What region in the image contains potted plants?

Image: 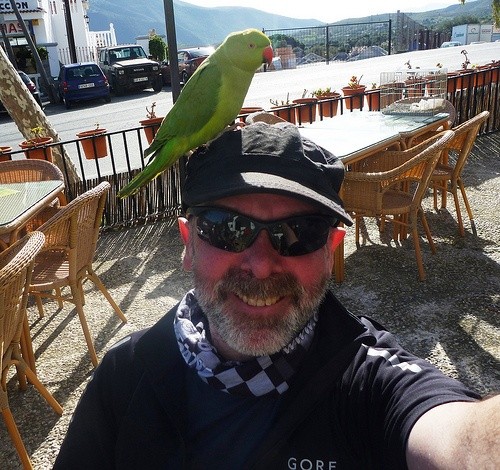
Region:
[269,93,297,125]
[139,101,165,146]
[294,89,317,123]
[311,87,340,117]
[76,123,109,160]
[20,125,54,164]
[366,83,382,111]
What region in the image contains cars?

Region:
[160,48,218,85]
[0,70,44,118]
[439,41,461,48]
[58,63,111,110]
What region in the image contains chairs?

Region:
[0,231,63,470]
[335,130,455,283]
[0,180,65,234]
[0,160,66,244]
[380,96,456,132]
[406,110,490,237]
[15,181,128,384]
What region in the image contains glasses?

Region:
[186,204,339,256]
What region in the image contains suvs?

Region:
[99,45,163,96]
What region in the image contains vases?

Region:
[342,86,366,109]
[456,70,475,89]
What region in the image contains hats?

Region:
[179,120,354,227]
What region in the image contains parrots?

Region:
[114,28,273,201]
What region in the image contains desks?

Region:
[271,111,449,210]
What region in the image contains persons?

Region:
[53,121,500,470]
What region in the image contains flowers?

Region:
[459,50,472,68]
[347,76,358,83]
